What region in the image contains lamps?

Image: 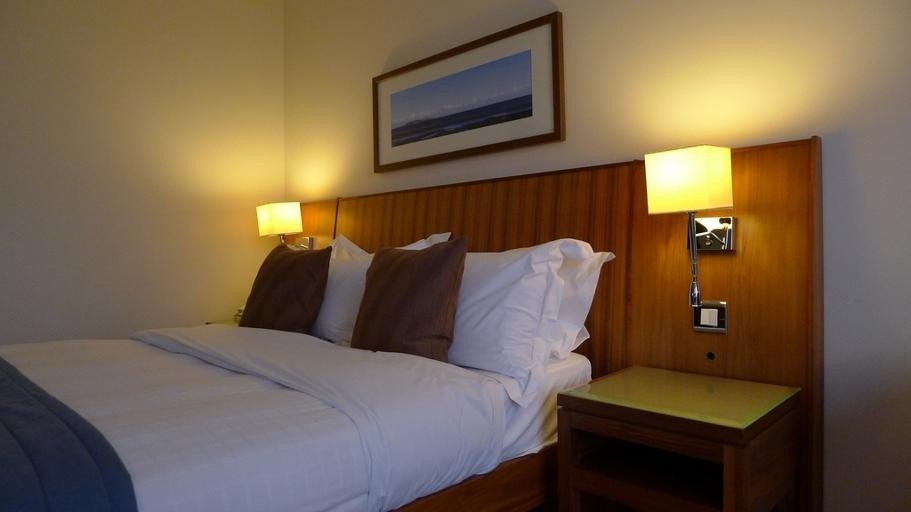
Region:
[642,143,735,312]
[255,201,312,253]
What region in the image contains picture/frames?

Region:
[371,10,566,173]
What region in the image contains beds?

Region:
[0,323,593,512]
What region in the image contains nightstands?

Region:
[551,354,801,512]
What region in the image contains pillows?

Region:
[310,236,450,341]
[236,243,333,334]
[449,239,618,408]
[350,236,466,363]
